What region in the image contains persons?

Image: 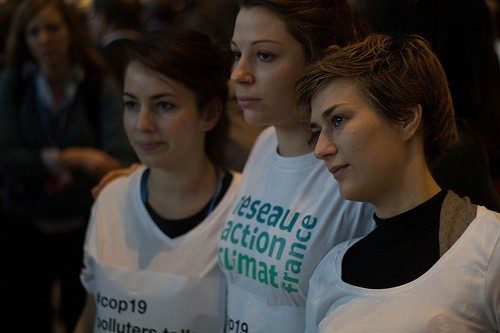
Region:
[0,1,242,333]
[215,0,376,333]
[297,28,500,333]
